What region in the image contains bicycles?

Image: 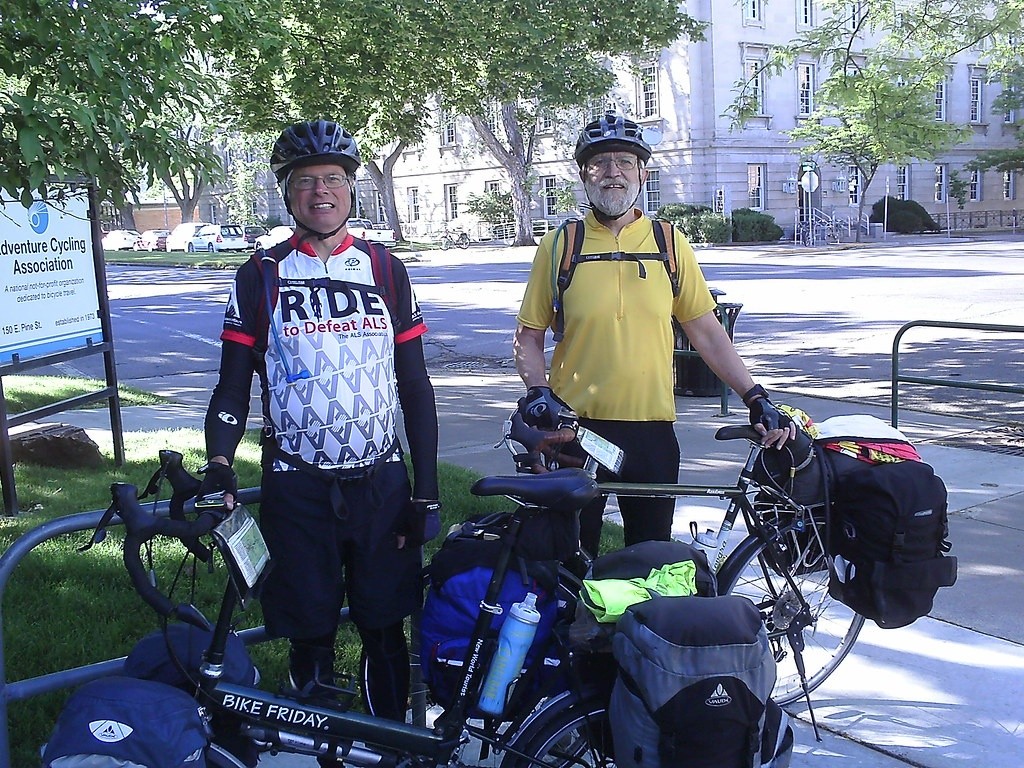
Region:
[77,447,618,768]
[359,424,867,746]
[438,229,470,250]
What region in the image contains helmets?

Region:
[575,116,652,170]
[270,120,362,183]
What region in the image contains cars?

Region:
[99,229,142,249]
[184,224,249,253]
[165,223,212,253]
[254,226,296,251]
[133,230,171,253]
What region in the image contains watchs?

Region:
[742,384,770,405]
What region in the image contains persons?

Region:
[197,118,440,768]
[514,114,796,559]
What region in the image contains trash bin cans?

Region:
[670,302,743,397]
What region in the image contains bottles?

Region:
[475,593,540,714]
[688,521,720,571]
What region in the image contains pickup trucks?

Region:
[345,218,396,249]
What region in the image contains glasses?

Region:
[290,174,350,190]
[585,156,638,170]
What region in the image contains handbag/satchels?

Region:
[41,675,212,768]
[608,595,793,768]
[753,447,869,577]
[128,623,261,768]
[828,460,957,629]
[570,540,718,757]
[420,511,592,729]
[775,403,922,464]
